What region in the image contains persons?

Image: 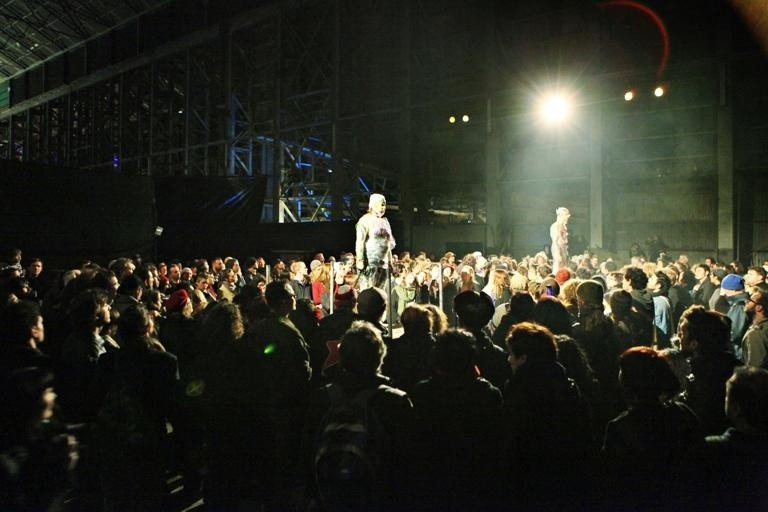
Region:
[355,192,397,289]
[1,234,768,511]
[549,206,571,274]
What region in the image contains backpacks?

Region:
[313,383,380,510]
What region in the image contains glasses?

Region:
[746,297,757,304]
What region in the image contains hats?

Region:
[576,280,603,304]
[165,289,187,311]
[359,287,385,314]
[452,291,494,326]
[720,275,746,290]
[611,290,633,313]
[332,284,357,307]
